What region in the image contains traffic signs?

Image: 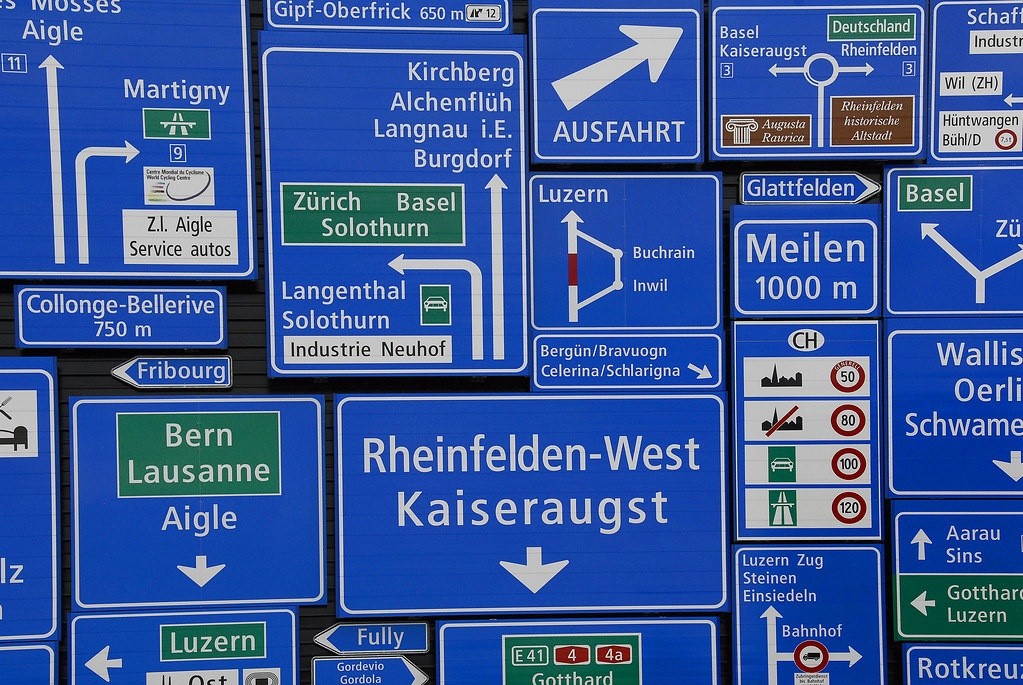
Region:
[67,393,328,613]
[528,171,727,393]
[261,0,513,34]
[892,500,1023,642]
[310,656,430,685]
[883,318,1023,499]
[901,642,1023,685]
[254,33,527,378]
[13,284,229,351]
[65,612,300,685]
[733,322,882,541]
[0,644,60,685]
[882,165,1022,317]
[434,618,723,685]
[927,0,1023,165]
[706,1,927,163]
[312,620,429,655]
[0,356,62,641]
[109,354,235,389]
[335,393,733,618]
[0,0,257,281]
[730,544,889,685]
[738,170,883,203]
[729,203,883,319]
[528,1,703,162]
